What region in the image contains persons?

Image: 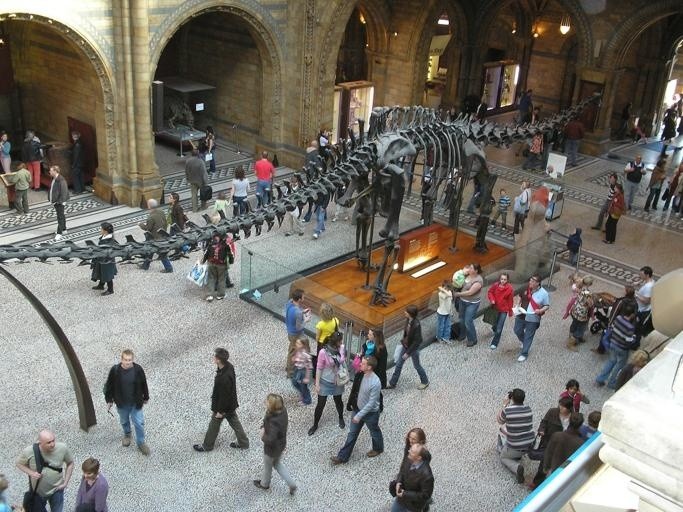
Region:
[567,266,655,392]
[436,263,550,363]
[284,289,388,463]
[74,458,108,511]
[497,380,601,490]
[284,127,349,238]
[590,103,683,244]
[392,428,435,510]
[1,430,74,510]
[385,305,431,390]
[421,166,533,239]
[513,90,585,172]
[253,394,296,495]
[569,227,583,265]
[102,350,150,454]
[193,348,250,452]
[0,127,276,305]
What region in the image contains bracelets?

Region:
[503,405,506,407]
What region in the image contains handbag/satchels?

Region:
[200,186,212,201]
[482,307,498,325]
[662,188,669,200]
[337,369,349,386]
[528,449,545,460]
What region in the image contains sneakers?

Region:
[226,283,234,287]
[313,234,317,238]
[418,381,430,389]
[332,217,339,222]
[122,433,131,446]
[345,216,349,221]
[92,287,104,289]
[55,230,68,235]
[490,345,497,349]
[206,296,213,301]
[253,480,269,489]
[230,441,242,448]
[521,343,524,348]
[138,262,149,270]
[290,486,297,494]
[55,234,63,241]
[193,444,213,451]
[160,269,173,273]
[528,483,538,489]
[367,450,381,457]
[517,465,524,484]
[436,335,478,346]
[331,456,341,462]
[102,289,113,296]
[216,296,225,299]
[138,443,150,455]
[517,356,526,361]
[386,382,395,389]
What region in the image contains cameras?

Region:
[508,391,513,399]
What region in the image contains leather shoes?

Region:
[308,424,318,435]
[339,417,345,428]
[508,233,514,237]
